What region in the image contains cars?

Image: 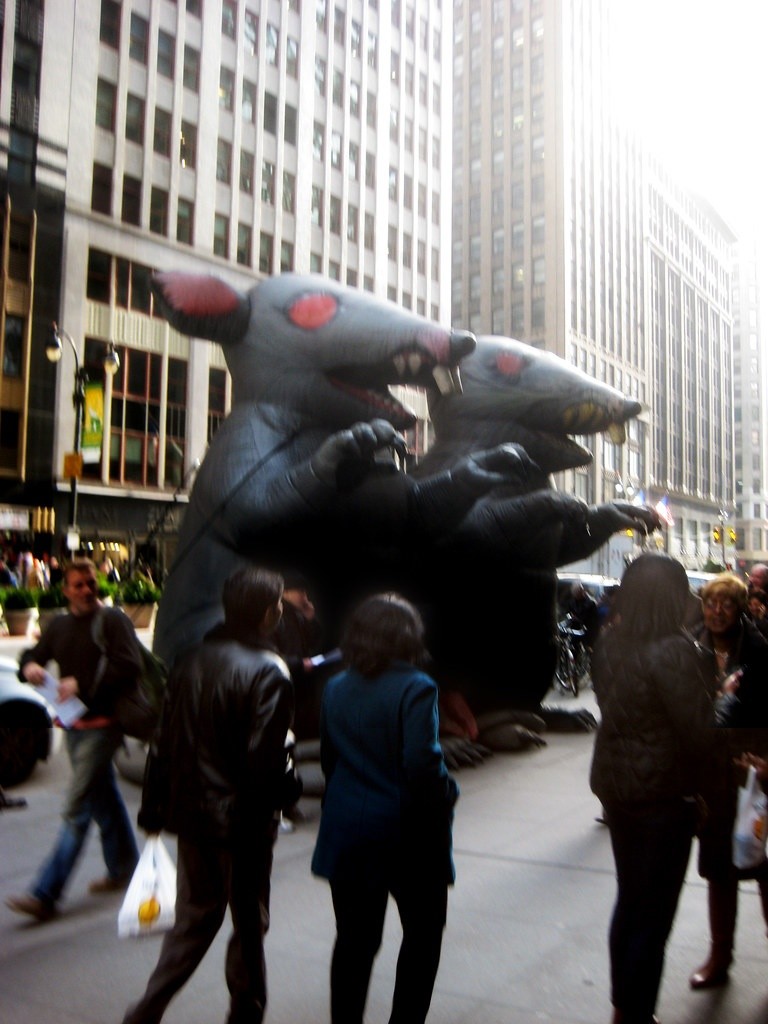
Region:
[0,655,56,789]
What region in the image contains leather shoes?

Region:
[86,876,130,894]
[3,895,59,921]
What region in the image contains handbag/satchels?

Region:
[731,765,768,868]
[117,834,178,940]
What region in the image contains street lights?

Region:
[718,509,729,568]
[47,322,120,564]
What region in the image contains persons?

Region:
[560,580,619,649]
[123,567,316,1024]
[4,559,144,919]
[680,554,768,988]
[309,595,461,1024]
[590,553,739,1024]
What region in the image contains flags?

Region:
[634,490,645,504]
[657,496,674,525]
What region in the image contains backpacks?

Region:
[91,605,171,743]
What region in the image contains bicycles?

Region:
[554,619,587,697]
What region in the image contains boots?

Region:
[690,943,734,989]
